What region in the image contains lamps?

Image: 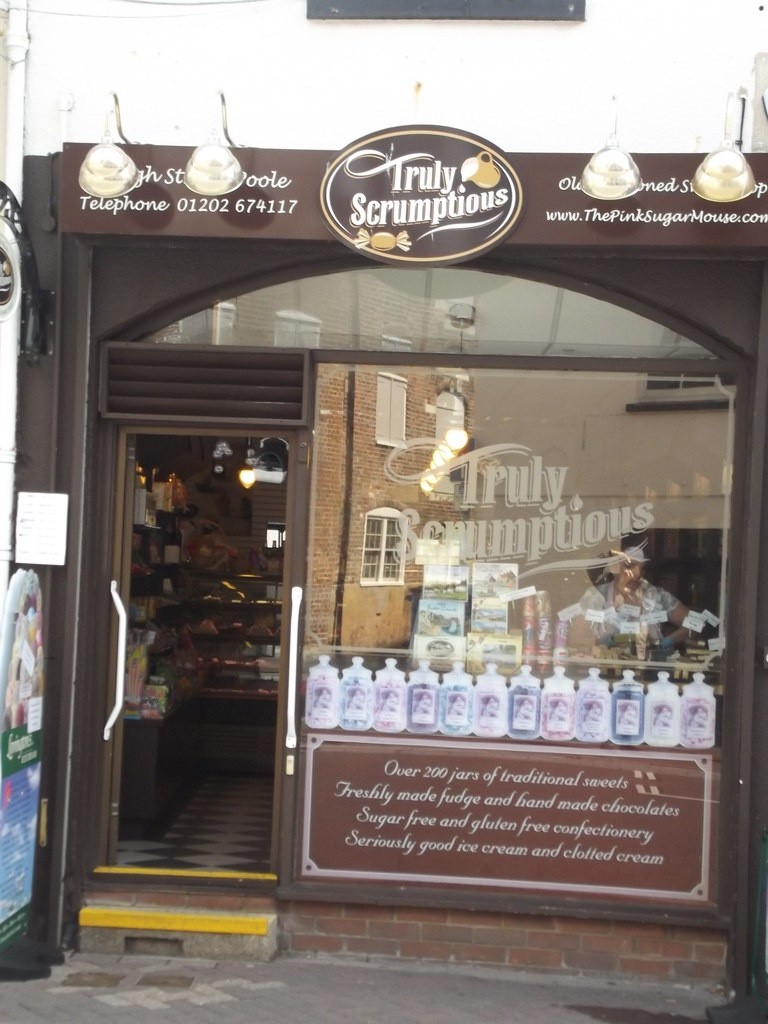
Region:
[580,95,645,201]
[687,89,756,203]
[240,437,257,490]
[77,94,141,198]
[182,90,247,196]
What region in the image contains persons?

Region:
[579,544,695,655]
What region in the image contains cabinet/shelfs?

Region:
[119,525,284,844]
